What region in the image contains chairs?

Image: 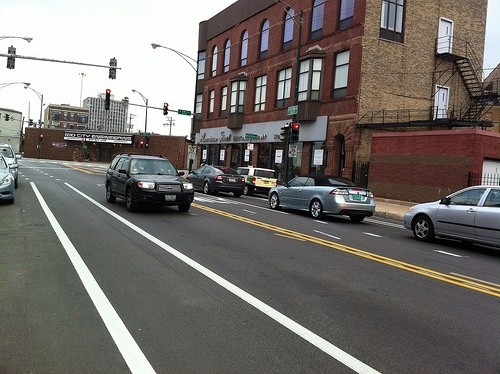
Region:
[487,192,496,202]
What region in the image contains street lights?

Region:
[151,43,200,174]
[25,86,43,128]
[132,90,148,132]
[79,73,86,108]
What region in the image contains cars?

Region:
[186,165,245,197]
[0,144,22,188]
[0,153,19,204]
[268,175,375,223]
[403,186,500,247]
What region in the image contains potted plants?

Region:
[83,153,90,162]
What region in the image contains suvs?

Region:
[236,167,278,196]
[106,152,193,212]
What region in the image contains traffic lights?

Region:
[40,135,43,141]
[147,138,150,143]
[290,123,300,142]
[6,114,9,121]
[105,89,111,110]
[280,124,290,144]
[163,103,169,115]
[135,141,139,149]
[141,141,144,149]
